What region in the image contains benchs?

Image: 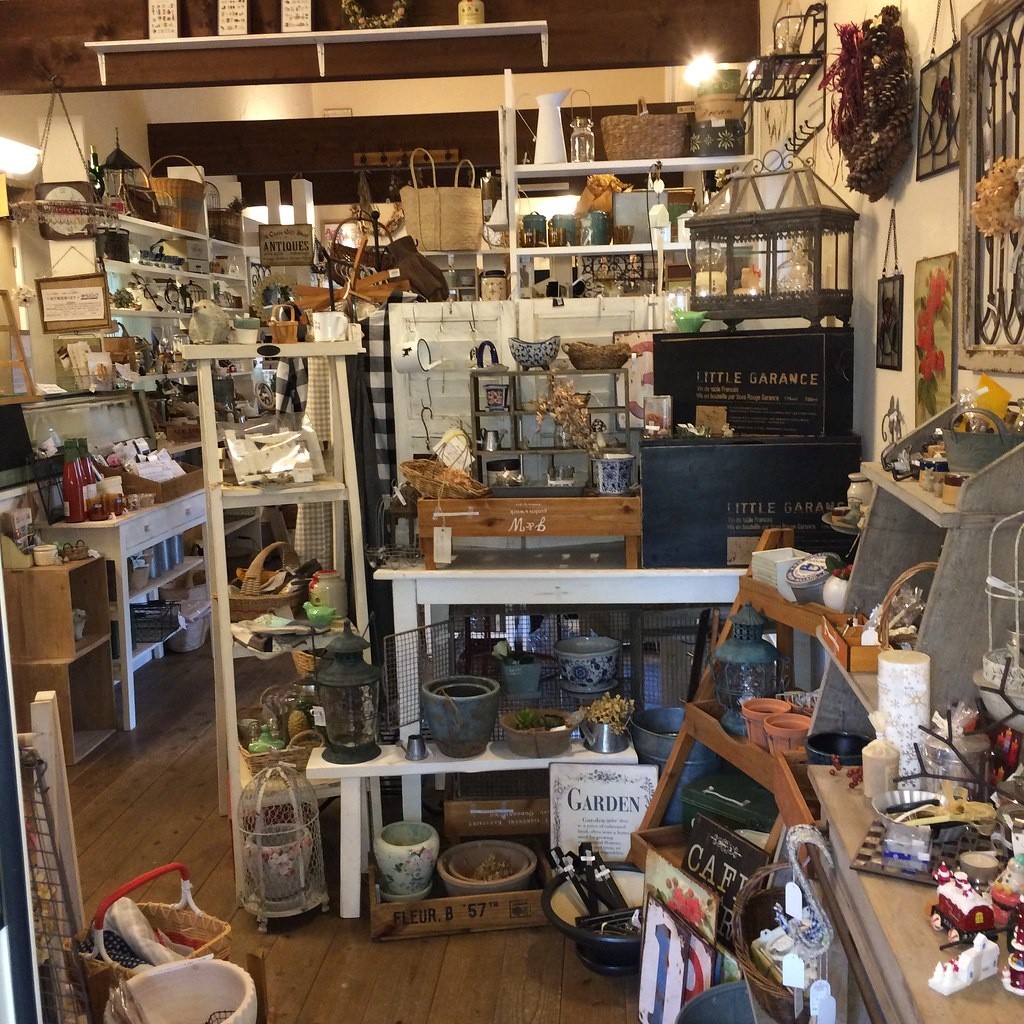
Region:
[307,732,638,917]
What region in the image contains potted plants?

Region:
[499,709,576,756]
[577,693,634,752]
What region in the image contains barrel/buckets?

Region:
[941,409,1024,473]
[632,709,724,829]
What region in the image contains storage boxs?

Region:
[751,546,812,587]
[96,462,204,503]
[682,152,861,331]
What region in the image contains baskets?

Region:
[327,217,394,289]
[210,542,312,622]
[731,862,816,1024]
[268,305,298,344]
[601,98,690,161]
[290,650,322,680]
[61,862,232,1024]
[149,155,206,232]
[236,744,314,779]
[877,563,939,653]
[400,433,493,503]
[60,539,88,560]
[131,599,183,643]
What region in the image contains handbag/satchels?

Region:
[103,321,138,373]
[376,235,450,302]
[399,148,482,251]
[119,161,162,223]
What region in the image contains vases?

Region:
[246,823,313,899]
[436,838,538,899]
[765,714,811,757]
[742,697,793,746]
[374,824,439,895]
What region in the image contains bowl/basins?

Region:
[508,336,561,370]
[871,789,950,836]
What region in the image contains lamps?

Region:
[1,134,43,175]
[712,602,792,736]
[97,123,150,214]
[314,619,383,766]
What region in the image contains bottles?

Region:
[62,437,96,522]
[87,145,105,201]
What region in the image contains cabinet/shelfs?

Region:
[0,64,1023,1023]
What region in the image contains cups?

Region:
[547,227,573,246]
[677,211,696,242]
[32,544,59,565]
[651,221,671,243]
[101,493,124,515]
[85,497,110,521]
[394,331,431,374]
[311,311,348,341]
[612,225,634,244]
[518,228,545,248]
